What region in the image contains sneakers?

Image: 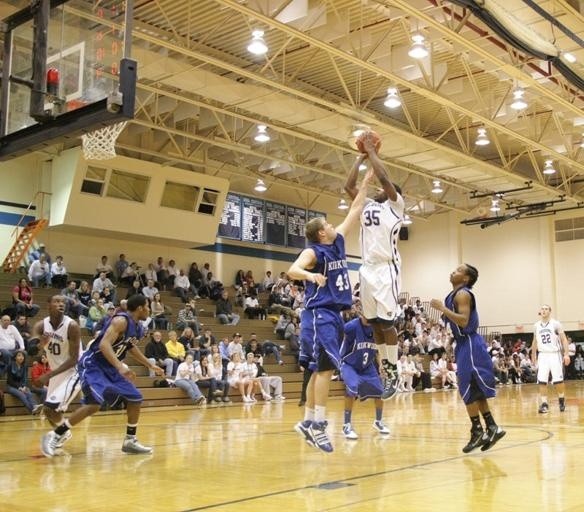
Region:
[195,393,286,404]
[396,384,450,393]
[342,422,358,439]
[481,424,506,451]
[31,404,43,414]
[538,402,549,413]
[381,358,402,401]
[294,419,316,448]
[462,423,486,453]
[307,420,333,452]
[56,429,72,448]
[121,434,152,454]
[41,430,62,459]
[559,397,565,411]
[373,419,390,433]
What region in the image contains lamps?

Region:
[431,179,444,194]
[253,177,268,193]
[490,197,500,212]
[253,124,271,142]
[542,157,556,174]
[383,84,402,110]
[474,125,491,146]
[349,121,373,173]
[406,29,429,59]
[246,25,269,55]
[337,198,349,211]
[510,85,528,110]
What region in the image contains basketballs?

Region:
[356,131,382,155]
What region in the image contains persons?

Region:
[430,264,506,453]
[487,303,584,414]
[343,283,362,322]
[392,298,458,393]
[0,241,311,458]
[290,166,374,452]
[339,304,390,439]
[344,136,404,401]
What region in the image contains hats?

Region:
[233,332,242,337]
[492,350,500,355]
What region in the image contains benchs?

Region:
[0,263,444,414]
